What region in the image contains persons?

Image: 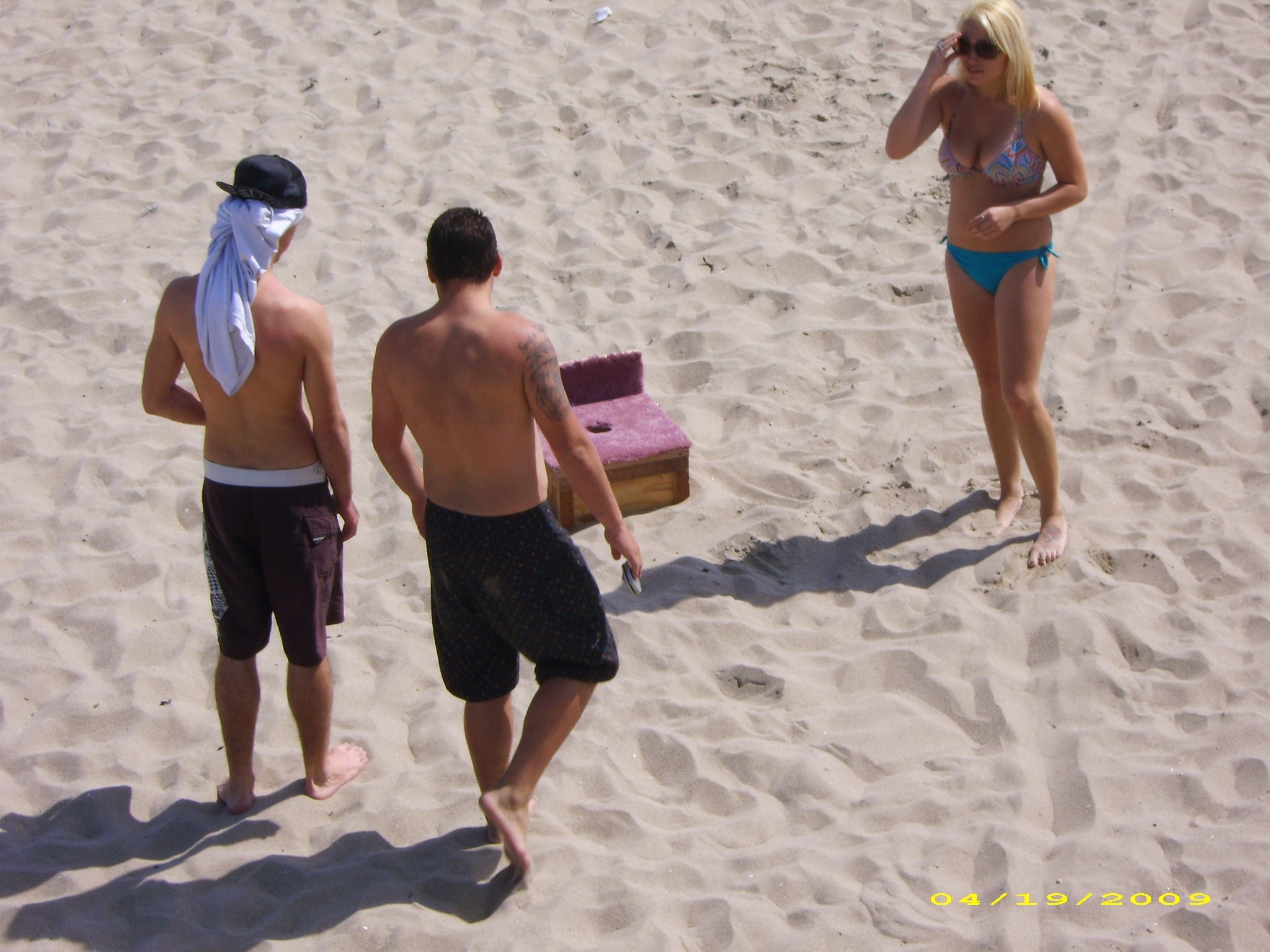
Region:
[886,0,1089,569]
[140,155,359,817]
[370,206,644,876]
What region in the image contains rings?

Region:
[979,213,985,220]
[935,36,945,53]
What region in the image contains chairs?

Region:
[543,350,693,528]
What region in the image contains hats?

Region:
[216,154,307,209]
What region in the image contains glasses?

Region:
[953,36,1004,60]
